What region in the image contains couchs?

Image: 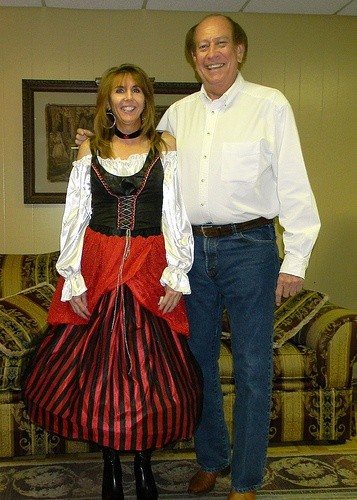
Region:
[0,250,357,461]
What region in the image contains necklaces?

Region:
[114,127,142,139]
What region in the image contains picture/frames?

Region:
[21,78,201,204]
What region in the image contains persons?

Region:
[27,62,204,500]
[74,14,321,500]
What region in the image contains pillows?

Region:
[0,281,56,358]
[222,287,327,350]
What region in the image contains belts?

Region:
[192,217,274,238]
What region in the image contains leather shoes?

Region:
[228,488,256,500]
[187,465,231,494]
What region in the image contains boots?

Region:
[102,452,124,500]
[134,450,158,500]
